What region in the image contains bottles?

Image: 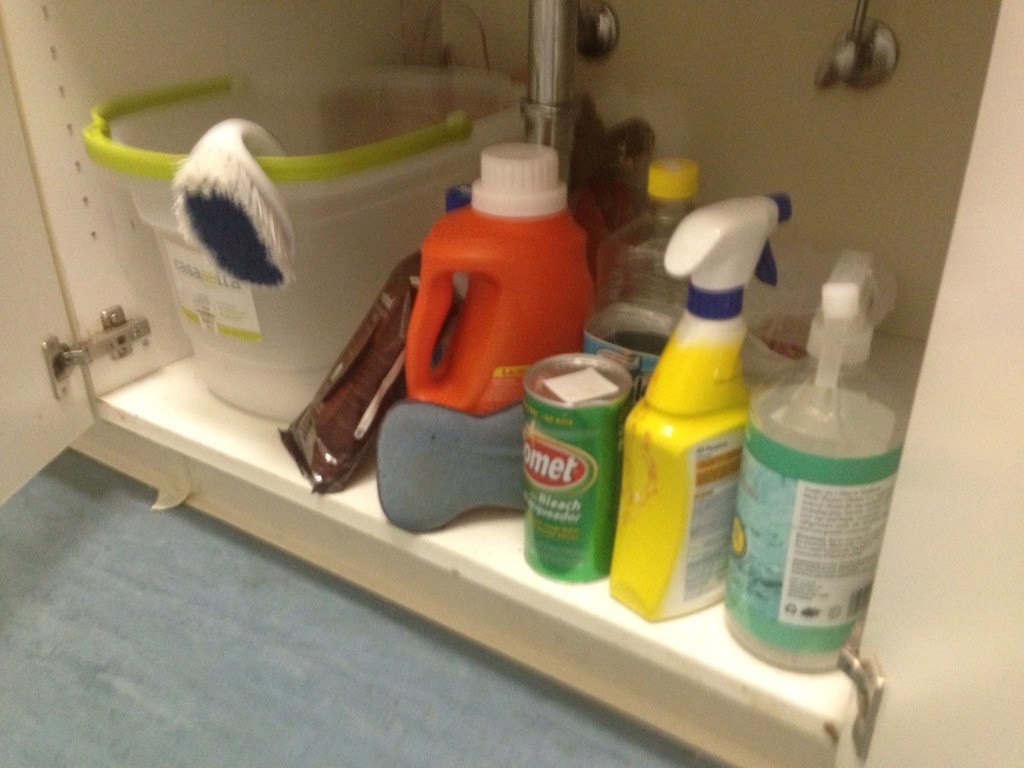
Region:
[608,188,792,622]
[724,244,907,672]
[581,158,709,404]
[408,141,598,422]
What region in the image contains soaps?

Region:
[375,397,526,536]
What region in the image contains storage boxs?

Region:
[78,61,532,427]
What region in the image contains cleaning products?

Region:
[402,140,915,674]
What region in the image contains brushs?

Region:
[168,114,297,290]
